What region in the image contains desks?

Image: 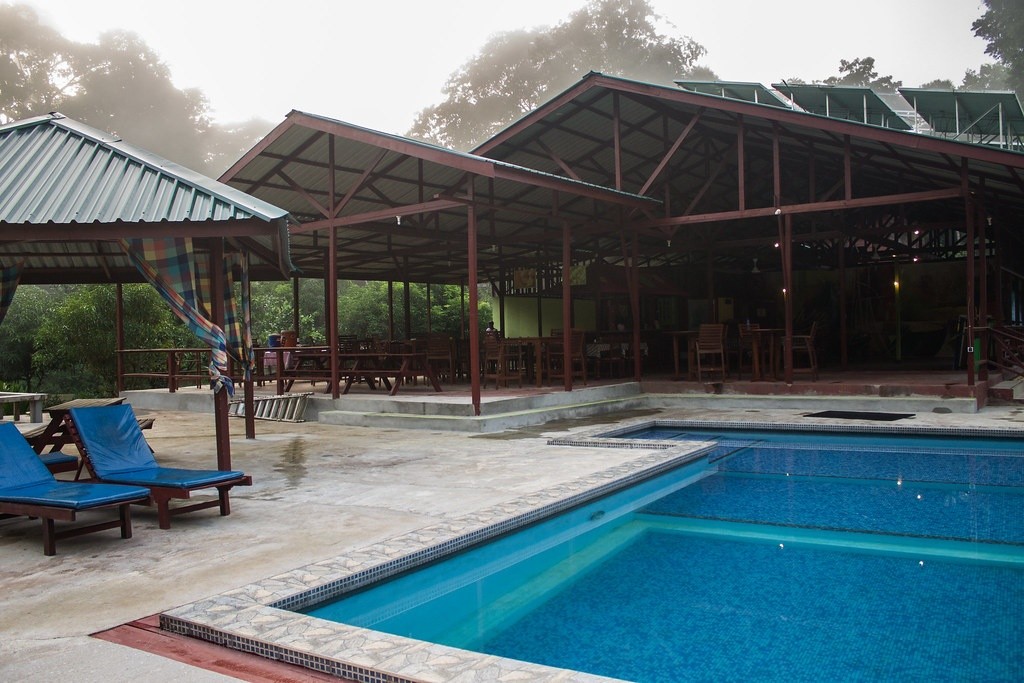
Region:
[292,354,443,396]
[751,328,786,383]
[664,330,698,380]
[34,397,154,456]
[504,336,560,386]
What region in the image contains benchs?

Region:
[283,369,402,375]
[347,367,443,374]
[137,418,155,431]
[23,423,50,447]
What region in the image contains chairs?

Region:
[236,327,634,390]
[38,451,78,476]
[64,404,252,530]
[0,422,150,555]
[692,320,823,383]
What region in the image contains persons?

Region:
[485,321,500,340]
[617,321,628,331]
[643,310,660,330]
